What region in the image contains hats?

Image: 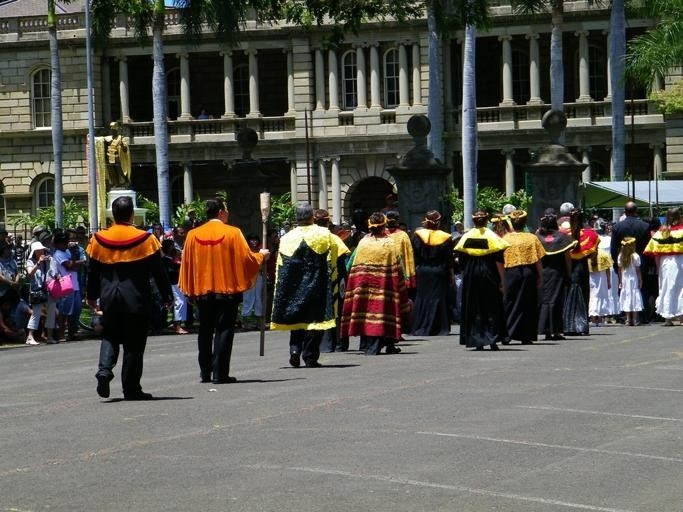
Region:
[28,241,47,258]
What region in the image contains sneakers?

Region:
[302,354,321,367]
[176,328,188,334]
[124,390,152,400]
[46,339,59,344]
[201,375,211,382]
[661,322,674,326]
[26,336,40,346]
[625,321,631,326]
[385,347,401,354]
[633,321,640,325]
[289,351,300,368]
[476,332,590,351]
[213,376,237,384]
[96,375,110,398]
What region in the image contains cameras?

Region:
[44,256,48,260]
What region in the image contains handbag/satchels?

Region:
[29,285,48,304]
[47,273,74,300]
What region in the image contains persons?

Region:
[84,195,173,402]
[0,210,265,346]
[267,201,682,370]
[177,197,270,384]
